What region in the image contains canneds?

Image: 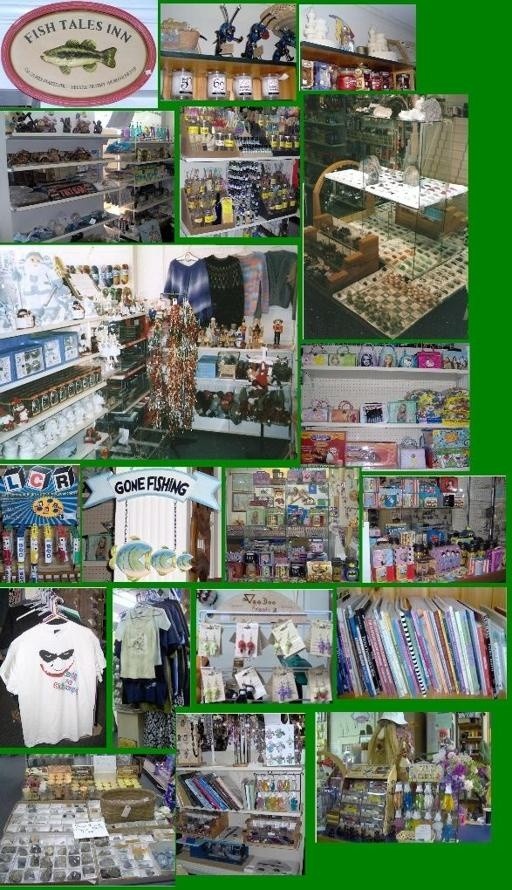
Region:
[170,66,281,100]
[260,173,297,215]
[48,774,143,800]
[380,73,394,90]
[254,114,300,151]
[188,119,234,151]
[183,177,224,227]
[397,73,410,90]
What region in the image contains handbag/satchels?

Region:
[395,434,427,471]
[400,348,419,367]
[379,344,399,366]
[331,399,359,423]
[301,400,329,423]
[328,346,357,367]
[302,343,328,367]
[416,343,442,368]
[360,401,390,424]
[442,350,467,369]
[386,399,417,423]
[356,342,379,367]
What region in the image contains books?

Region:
[336,590,507,700]
[176,771,255,811]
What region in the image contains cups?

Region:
[301,63,410,92]
[356,46,369,56]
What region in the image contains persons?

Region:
[381,353,392,366]
[395,403,409,423]
[360,353,374,367]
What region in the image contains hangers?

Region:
[119,590,179,619]
[11,589,91,634]
[172,245,298,269]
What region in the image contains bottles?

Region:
[123,121,170,141]
[170,68,281,101]
[255,779,298,812]
[186,107,299,159]
[394,780,456,843]
[412,530,496,577]
[185,161,297,232]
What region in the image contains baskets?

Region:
[100,789,157,824]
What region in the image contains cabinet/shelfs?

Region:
[318,759,488,845]
[305,95,468,339]
[179,107,300,238]
[363,477,506,582]
[301,344,470,471]
[228,467,359,580]
[159,50,296,100]
[1,112,174,243]
[300,40,416,91]
[177,713,305,874]
